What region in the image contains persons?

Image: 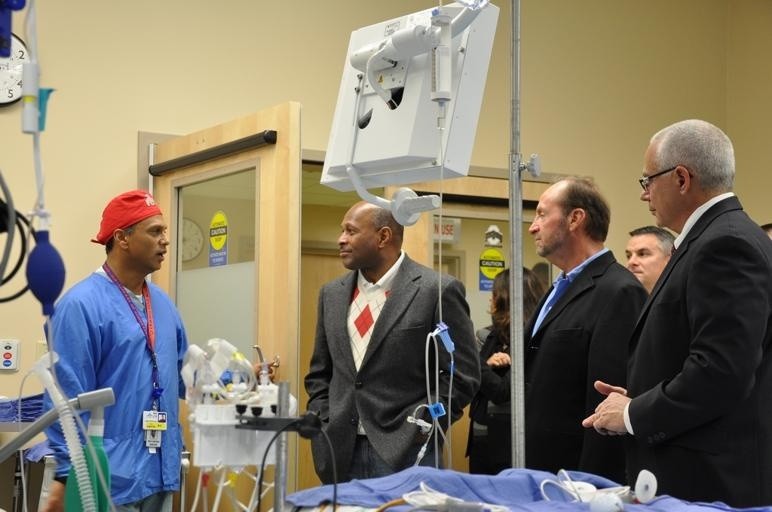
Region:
[465,176,677,489]
[582,119,771,509]
[42,190,276,512]
[304,201,481,486]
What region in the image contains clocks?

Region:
[0,30,31,109]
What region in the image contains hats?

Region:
[97,189,162,245]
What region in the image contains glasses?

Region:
[640,164,693,192]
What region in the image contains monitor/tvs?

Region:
[320,0,500,193]
